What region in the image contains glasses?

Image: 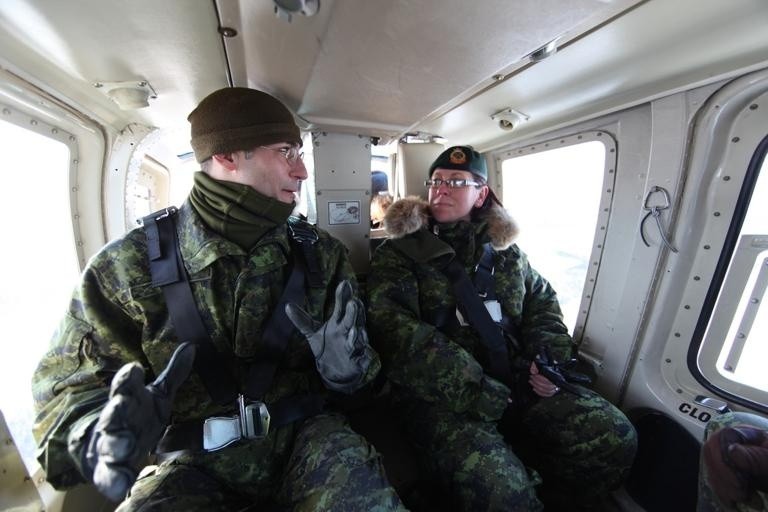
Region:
[260,146,303,165]
[424,178,479,188]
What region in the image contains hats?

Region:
[187,87,303,163]
[430,146,487,181]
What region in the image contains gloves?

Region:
[81,341,196,502]
[514,346,591,409]
[704,424,767,508]
[284,280,382,395]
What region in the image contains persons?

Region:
[366,146,640,512]
[33,86,411,512]
[698,412,767,512]
